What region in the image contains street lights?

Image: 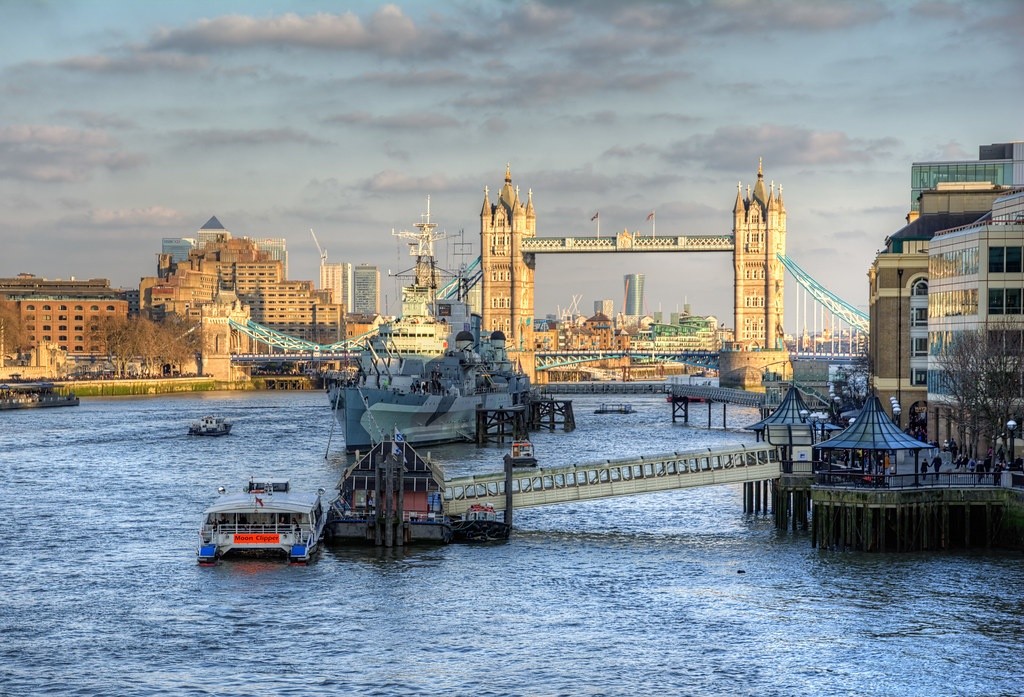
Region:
[810,412,819,444]
[819,415,828,461]
[1007,420,1017,467]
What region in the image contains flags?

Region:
[646,212,653,220]
[591,212,598,221]
[392,442,407,463]
[395,429,404,442]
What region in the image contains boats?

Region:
[322,193,541,456]
[0,382,80,411]
[325,427,449,544]
[195,474,324,565]
[186,414,235,438]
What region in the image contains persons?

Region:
[921,452,942,480]
[815,449,891,488]
[927,438,1024,488]
[211,514,300,531]
[902,414,928,456]
[410,379,442,396]
